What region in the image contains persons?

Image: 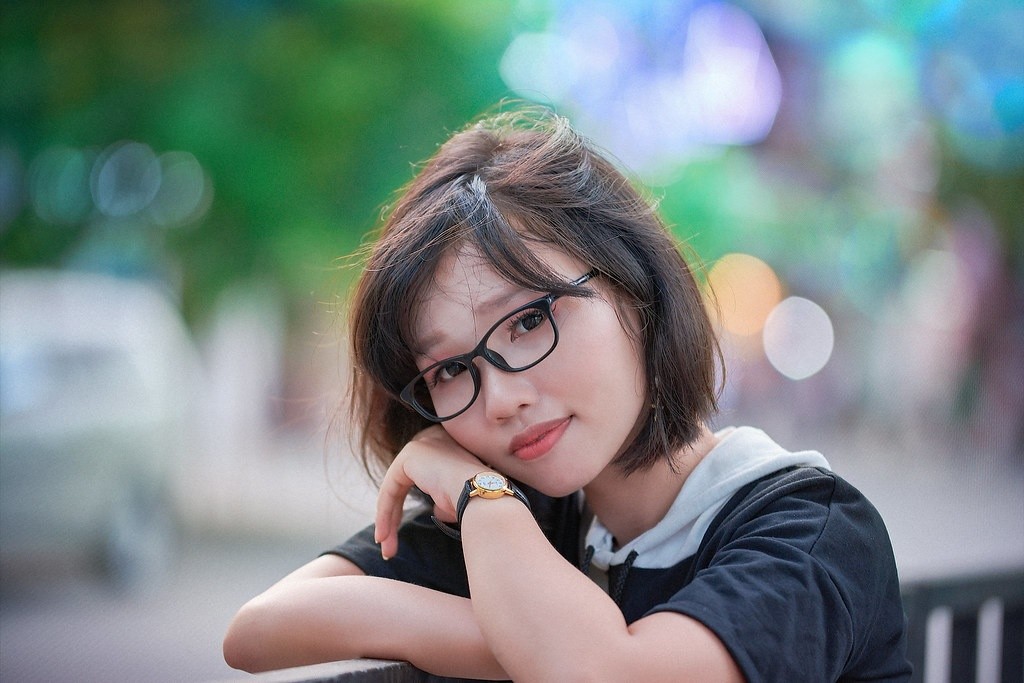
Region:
[222,97,918,683]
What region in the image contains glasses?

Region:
[400,269,603,423]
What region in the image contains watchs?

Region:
[455,471,531,535]
